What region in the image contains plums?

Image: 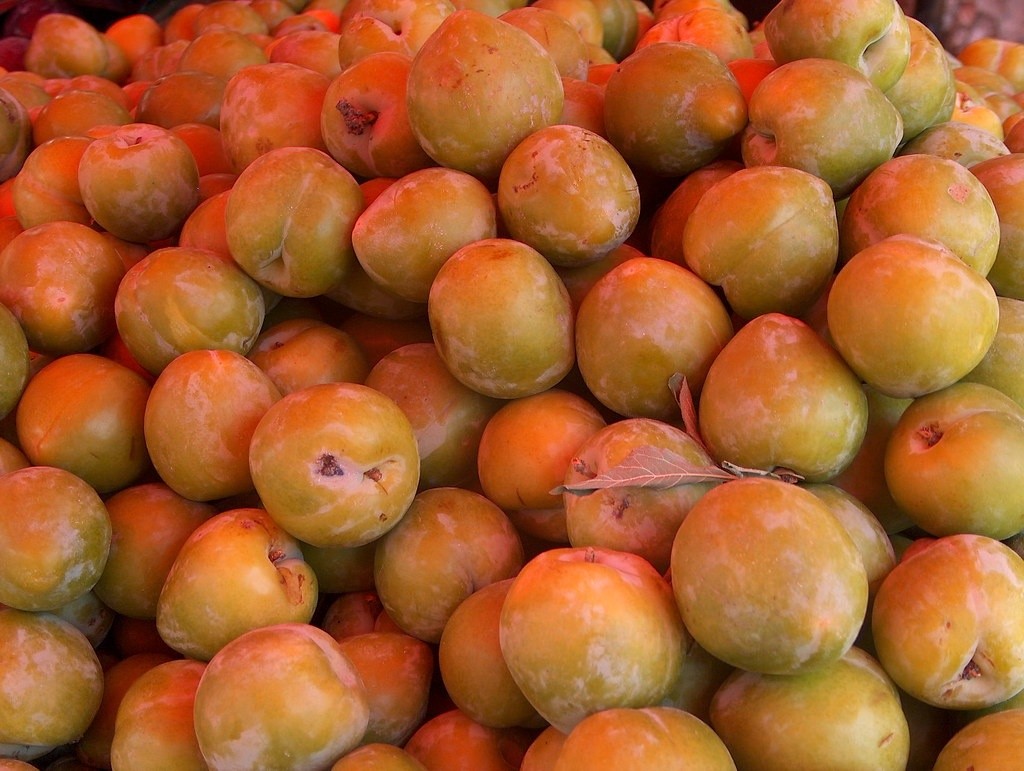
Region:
[0,0,1024,770]
